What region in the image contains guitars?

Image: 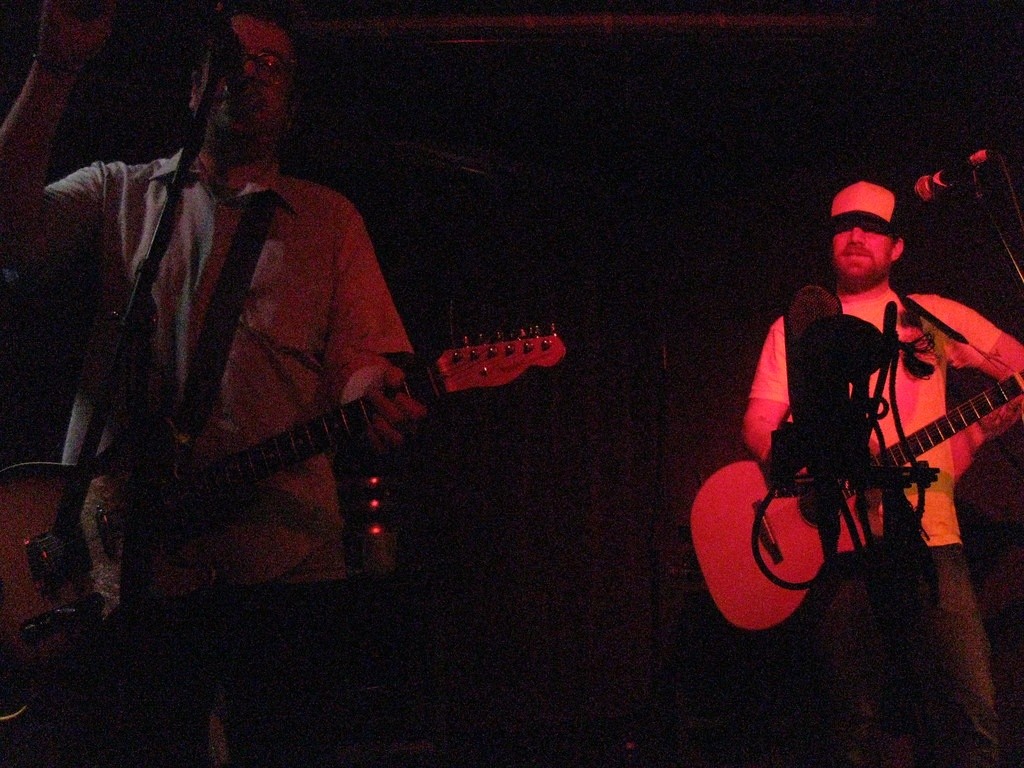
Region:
[686,364,1024,635]
[0,318,566,681]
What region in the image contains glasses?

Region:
[235,47,294,76]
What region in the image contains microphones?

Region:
[198,0,254,97]
[913,150,988,202]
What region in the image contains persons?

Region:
[0,0,432,768]
[740,181,1024,768]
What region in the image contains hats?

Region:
[831,181,897,224]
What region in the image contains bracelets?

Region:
[33,53,84,79]
